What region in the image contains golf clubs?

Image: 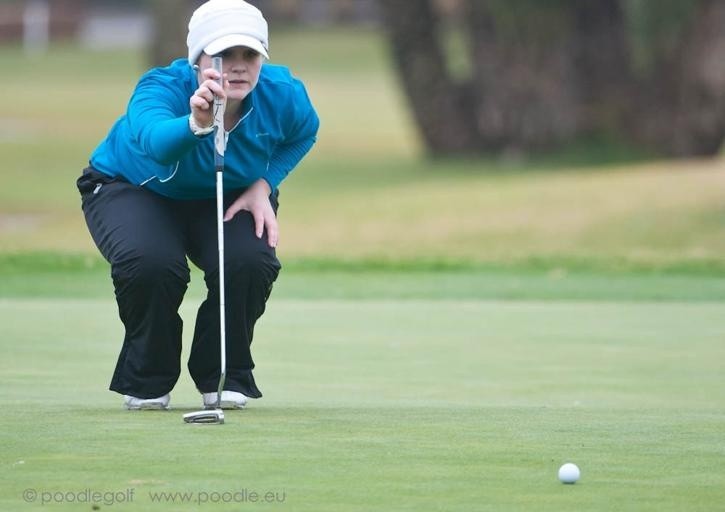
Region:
[182,54,227,425]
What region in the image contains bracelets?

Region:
[189,113,215,137]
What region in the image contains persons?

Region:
[76,0,319,411]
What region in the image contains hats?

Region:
[186,0,270,68]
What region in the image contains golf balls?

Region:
[557,463,580,484]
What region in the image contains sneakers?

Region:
[123,394,170,410]
[202,390,248,409]
